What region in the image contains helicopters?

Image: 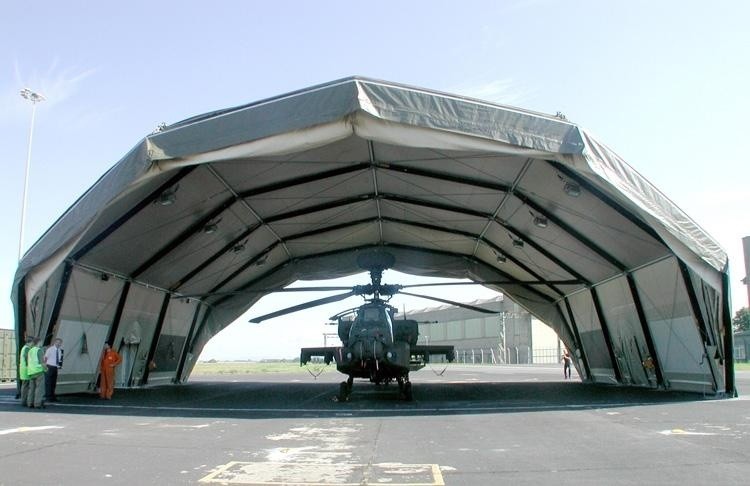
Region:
[166,253,586,402]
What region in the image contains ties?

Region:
[56,348,59,365]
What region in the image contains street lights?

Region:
[14,84,43,265]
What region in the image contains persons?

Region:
[98,340,120,401]
[26,337,48,409]
[560,348,572,380]
[43,336,64,403]
[18,335,33,405]
